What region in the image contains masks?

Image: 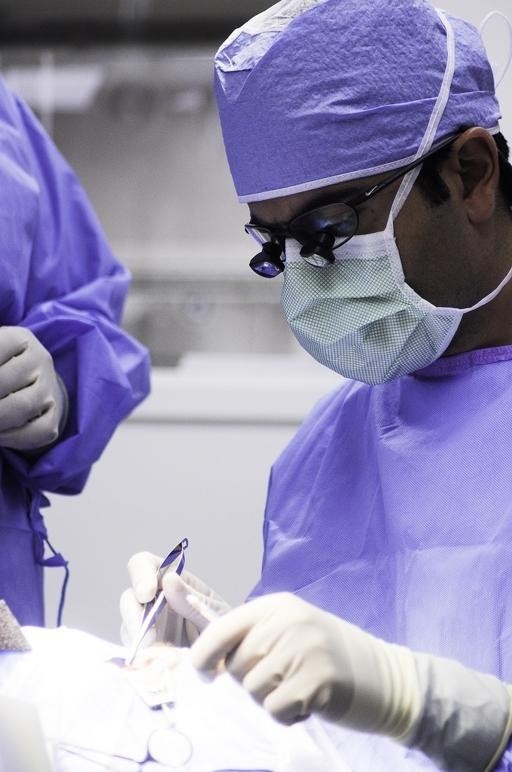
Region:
[280,150,468,385]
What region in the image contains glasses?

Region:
[244,132,458,252]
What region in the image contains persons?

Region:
[1,67,155,634]
[113,1,512,772]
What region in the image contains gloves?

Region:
[192,592,512,772]
[120,550,228,644]
[0,324,70,451]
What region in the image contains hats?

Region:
[214,0,500,201]
